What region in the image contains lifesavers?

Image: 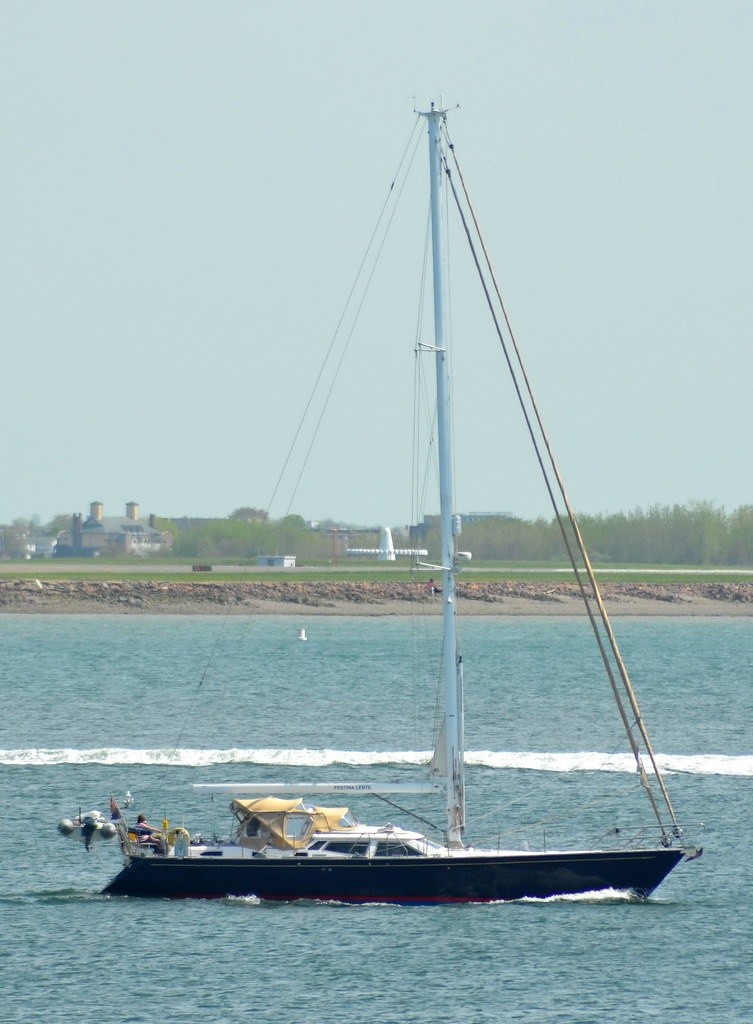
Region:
[169,827,189,846]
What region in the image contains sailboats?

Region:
[91,95,702,903]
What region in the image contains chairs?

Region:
[126,828,157,857]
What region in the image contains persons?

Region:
[135,814,162,852]
